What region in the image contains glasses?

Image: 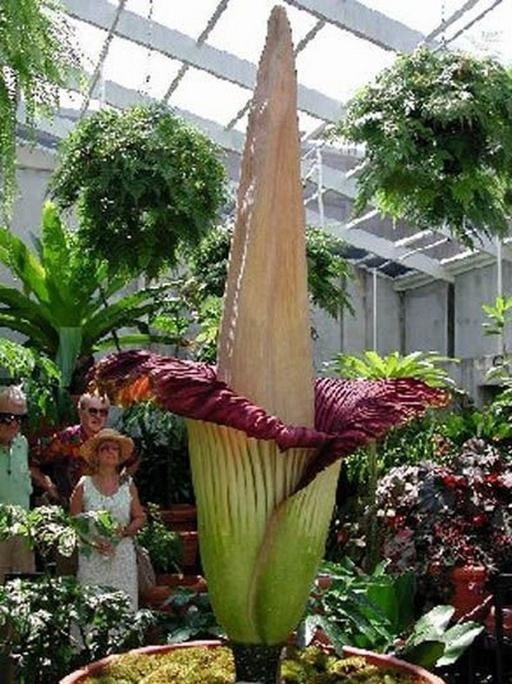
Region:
[0,410,28,426]
[83,406,109,415]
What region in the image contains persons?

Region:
[69,428,148,654]
[31,385,111,513]
[1,382,30,513]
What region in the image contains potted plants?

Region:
[0,0,512,684]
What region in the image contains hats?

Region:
[78,426,136,467]
